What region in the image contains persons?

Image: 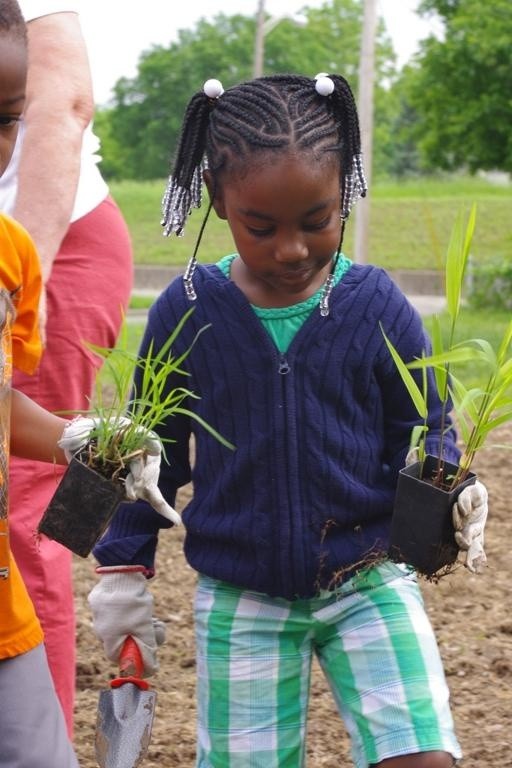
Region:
[86,71,492,768]
[0,1,136,744]
[1,1,185,768]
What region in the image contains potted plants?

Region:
[372,204,511,579]
[35,301,237,561]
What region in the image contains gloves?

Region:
[60,412,187,532]
[447,478,492,574]
[86,561,170,679]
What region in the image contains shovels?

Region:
[94,636,157,766]
